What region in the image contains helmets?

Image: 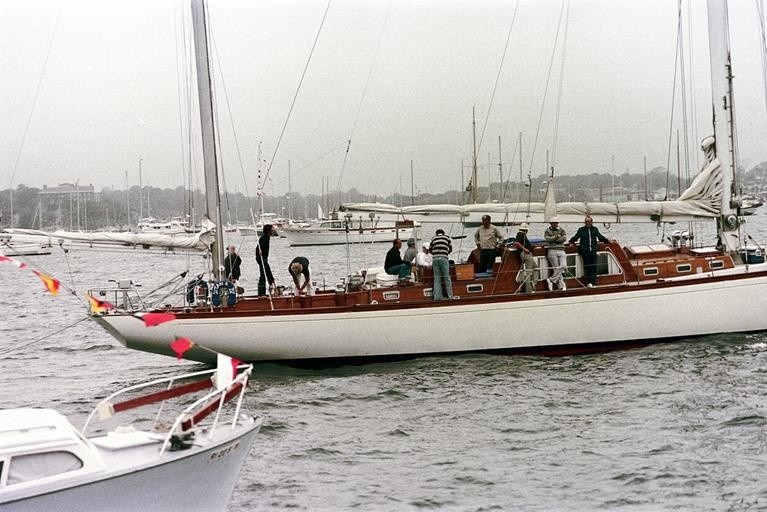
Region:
[518,222,529,230]
[548,217,559,223]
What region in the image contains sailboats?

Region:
[0,131,331,258]
[92,0,767,366]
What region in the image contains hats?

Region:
[407,238,415,245]
[423,242,431,250]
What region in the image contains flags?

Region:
[33,271,59,294]
[0,256,26,270]
[217,352,241,390]
[142,313,176,327]
[170,337,194,359]
[85,294,113,314]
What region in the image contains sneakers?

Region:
[546,278,553,291]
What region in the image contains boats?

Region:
[0,363,262,512]
[734,188,762,216]
[277,203,422,247]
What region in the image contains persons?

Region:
[289,256,315,297]
[384,227,460,301]
[474,215,504,272]
[515,223,538,294]
[255,224,275,299]
[568,216,616,289]
[544,217,567,292]
[225,246,242,284]
